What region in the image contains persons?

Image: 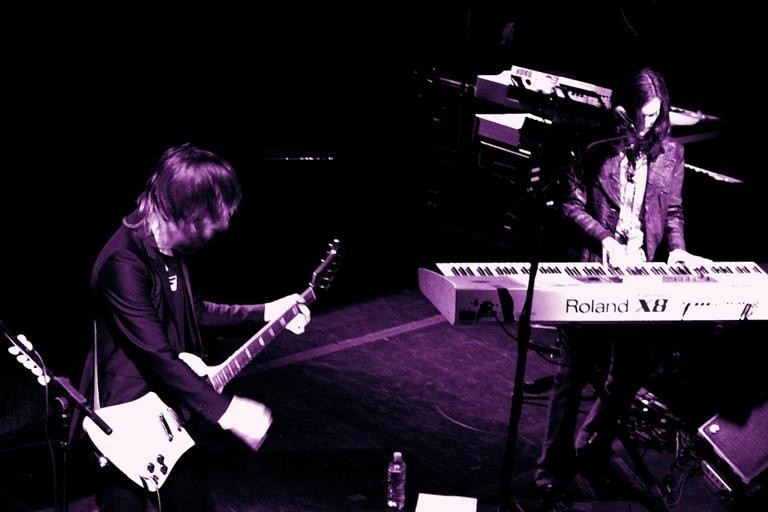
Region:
[531,69,715,502]
[65,144,312,508]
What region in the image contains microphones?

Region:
[613,106,637,136]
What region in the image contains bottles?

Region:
[387,452,406,512]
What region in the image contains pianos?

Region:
[418,261,767,326]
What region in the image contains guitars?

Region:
[80,239,342,493]
